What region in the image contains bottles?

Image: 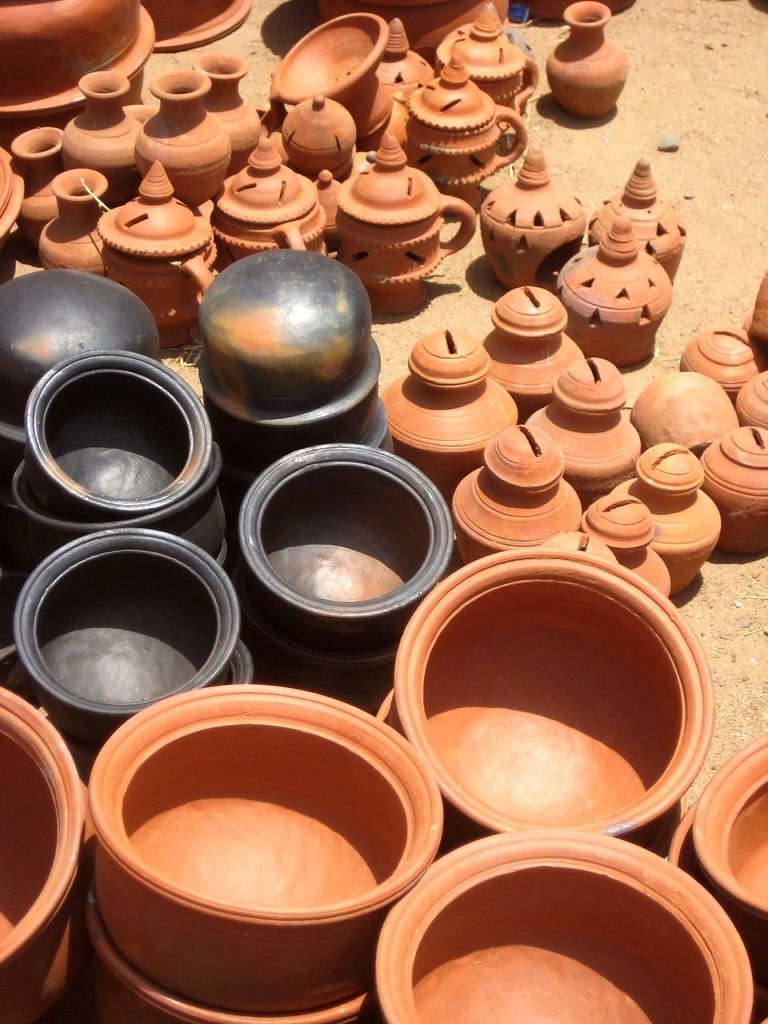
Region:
[0,52,261,278]
[546,1,630,118]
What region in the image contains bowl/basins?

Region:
[0,250,768,1024]
